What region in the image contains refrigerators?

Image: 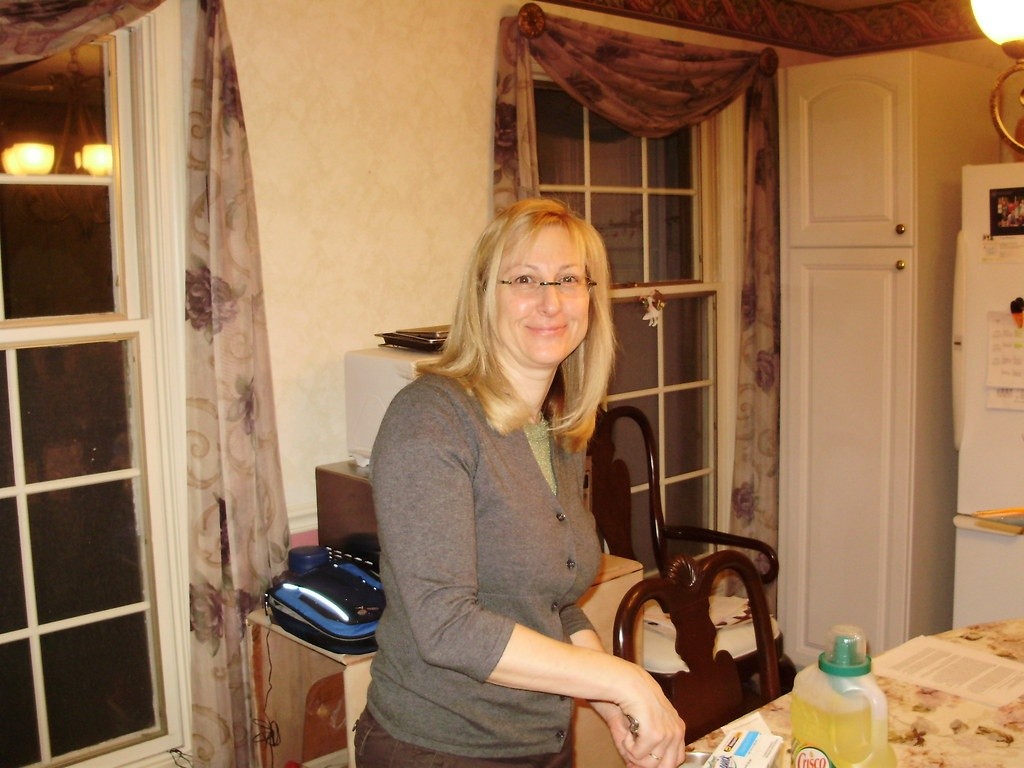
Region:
[951,164,1024,629]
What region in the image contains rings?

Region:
[649,753,663,760]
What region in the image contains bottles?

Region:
[791,624,898,768]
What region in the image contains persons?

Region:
[354,200,685,768]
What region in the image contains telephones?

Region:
[264,540,383,655]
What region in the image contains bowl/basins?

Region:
[677,752,712,768]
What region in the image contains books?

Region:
[642,595,775,642]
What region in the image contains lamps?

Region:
[958,0,1024,151]
[1,45,127,178]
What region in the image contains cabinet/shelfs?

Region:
[777,51,998,664]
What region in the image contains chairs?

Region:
[589,404,800,689]
[611,550,781,750]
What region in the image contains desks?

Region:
[671,618,1023,768]
[246,554,648,767]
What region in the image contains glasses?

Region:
[497,269,597,298]
